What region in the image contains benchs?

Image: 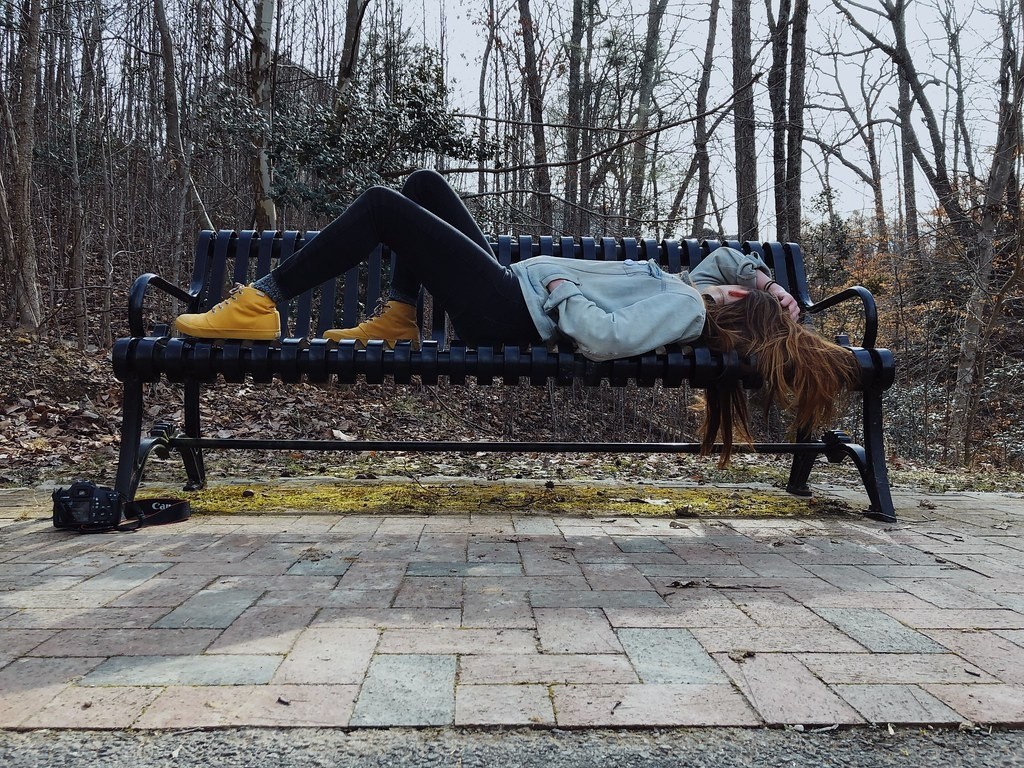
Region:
[113,230,897,523]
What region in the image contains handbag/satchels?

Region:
[51,480,190,534]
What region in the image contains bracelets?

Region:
[764,280,776,292]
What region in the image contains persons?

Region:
[171,169,857,472]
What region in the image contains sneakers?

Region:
[175,282,281,340]
[322,299,420,352]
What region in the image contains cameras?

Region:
[51,479,123,528]
